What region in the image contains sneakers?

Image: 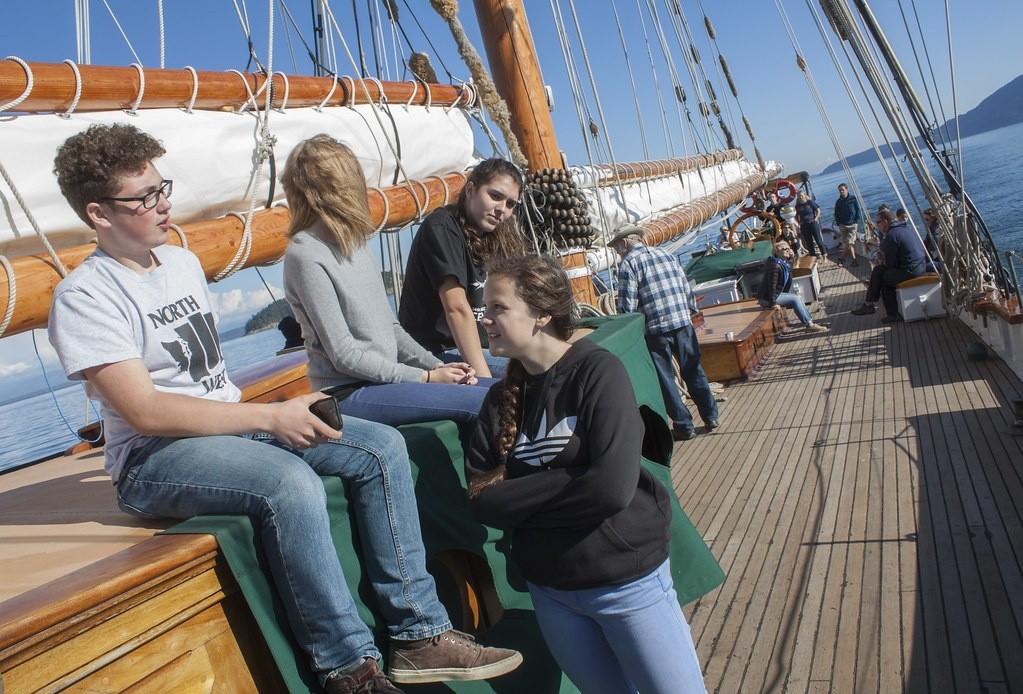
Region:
[387,627,525,684]
[881,313,903,323]
[322,657,406,694]
[851,302,875,315]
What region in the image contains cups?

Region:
[724,332,734,340]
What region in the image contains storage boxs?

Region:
[691,273,740,308]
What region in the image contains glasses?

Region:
[784,227,790,229]
[97,179,174,209]
[781,247,791,251]
[925,218,930,221]
[877,220,884,224]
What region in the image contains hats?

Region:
[606,222,644,248]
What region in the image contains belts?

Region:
[805,221,814,224]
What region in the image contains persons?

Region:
[851,204,944,323]
[776,223,800,255]
[756,241,828,332]
[466,256,705,694]
[795,191,827,263]
[280,133,501,424]
[47,122,524,694]
[606,223,719,439]
[766,193,786,228]
[834,183,859,266]
[399,157,524,378]
[717,226,740,252]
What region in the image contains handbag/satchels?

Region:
[794,237,808,257]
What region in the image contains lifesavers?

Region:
[771,179,797,203]
[736,191,758,213]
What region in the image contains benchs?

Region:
[869,261,946,322]
[793,256,820,305]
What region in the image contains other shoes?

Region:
[850,258,858,267]
[669,427,696,441]
[837,258,844,267]
[704,418,719,429]
[805,322,827,332]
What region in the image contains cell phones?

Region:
[309,397,343,432]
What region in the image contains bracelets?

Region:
[427,369,430,383]
